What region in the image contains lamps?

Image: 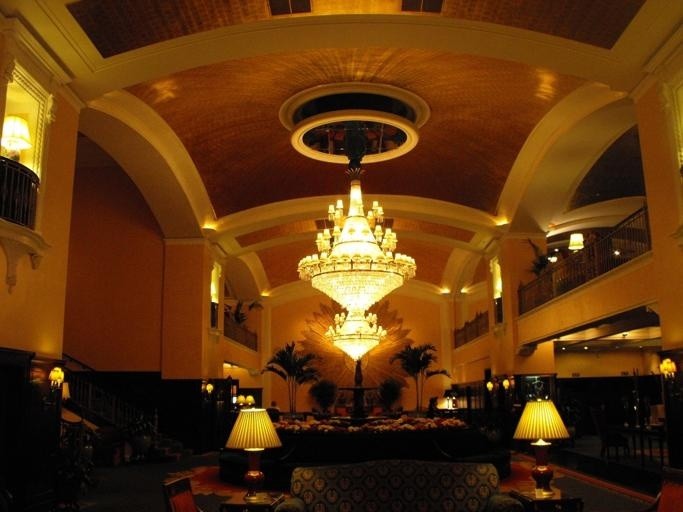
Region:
[659,358,683,398]
[41,367,71,408]
[566,233,586,255]
[224,406,284,500]
[512,400,571,495]
[0,115,32,162]
[278,82,432,362]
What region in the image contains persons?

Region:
[428,397,441,413]
[265,401,284,421]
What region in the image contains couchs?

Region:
[274,459,525,512]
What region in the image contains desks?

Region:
[221,492,285,512]
[509,486,585,512]
[603,424,666,470]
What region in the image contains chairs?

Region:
[634,478,683,512]
[163,477,203,512]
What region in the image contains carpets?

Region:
[162,450,657,512]
